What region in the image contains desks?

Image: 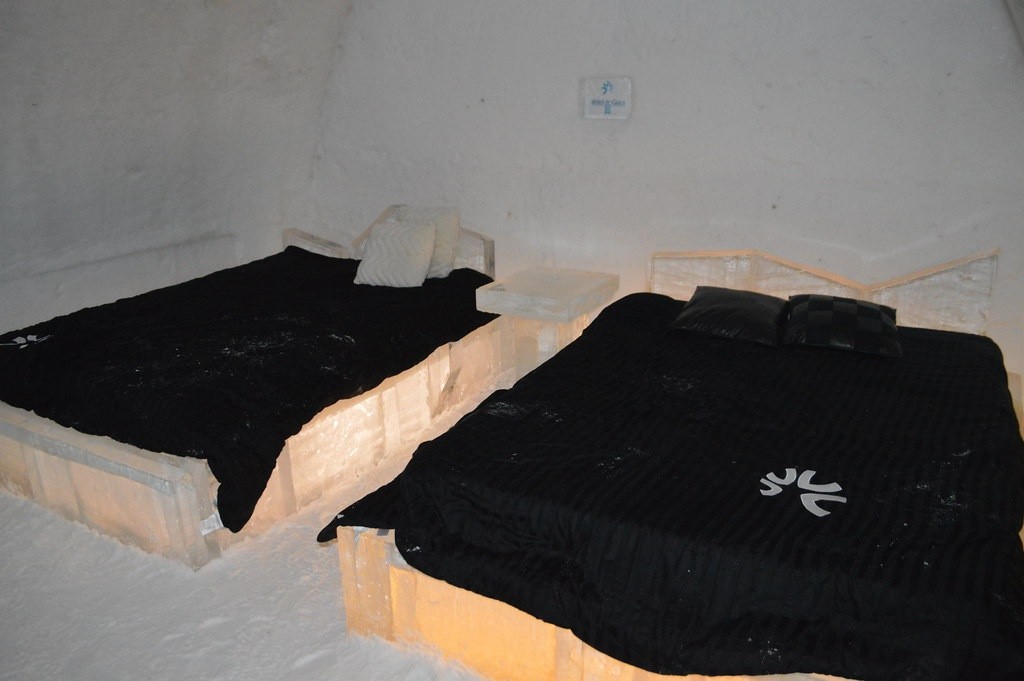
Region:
[475,266,619,375]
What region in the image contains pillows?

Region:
[353,222,436,288]
[666,285,790,347]
[391,206,461,281]
[783,294,903,358]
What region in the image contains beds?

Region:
[317,248,1024,681]
[0,205,516,570]
